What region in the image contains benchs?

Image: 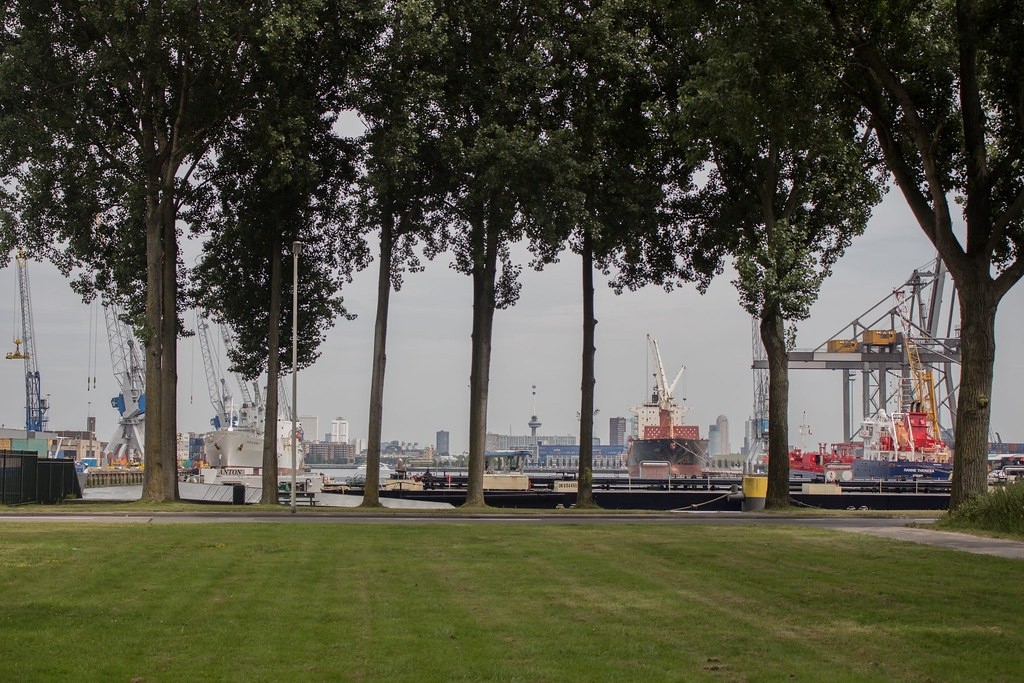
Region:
[278,491,320,507]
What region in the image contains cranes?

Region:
[0,239,56,434]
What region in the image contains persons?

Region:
[423,469,434,487]
